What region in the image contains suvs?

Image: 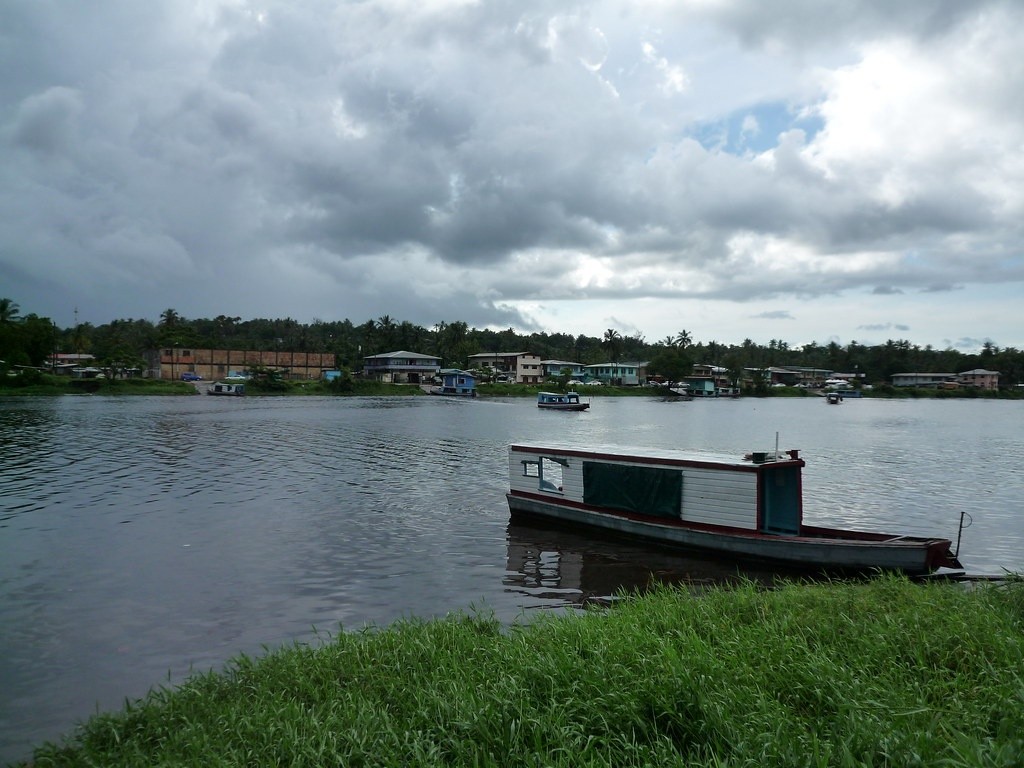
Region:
[181,372,203,382]
[835,382,854,390]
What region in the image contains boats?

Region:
[505,431,965,582]
[72,366,102,378]
[429,385,476,396]
[538,391,591,411]
[43,361,79,375]
[669,376,741,397]
[826,393,839,404]
[207,382,246,396]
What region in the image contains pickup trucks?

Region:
[642,381,661,388]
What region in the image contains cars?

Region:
[567,379,584,386]
[225,375,246,381]
[675,382,690,388]
[585,380,601,386]
[662,381,676,388]
